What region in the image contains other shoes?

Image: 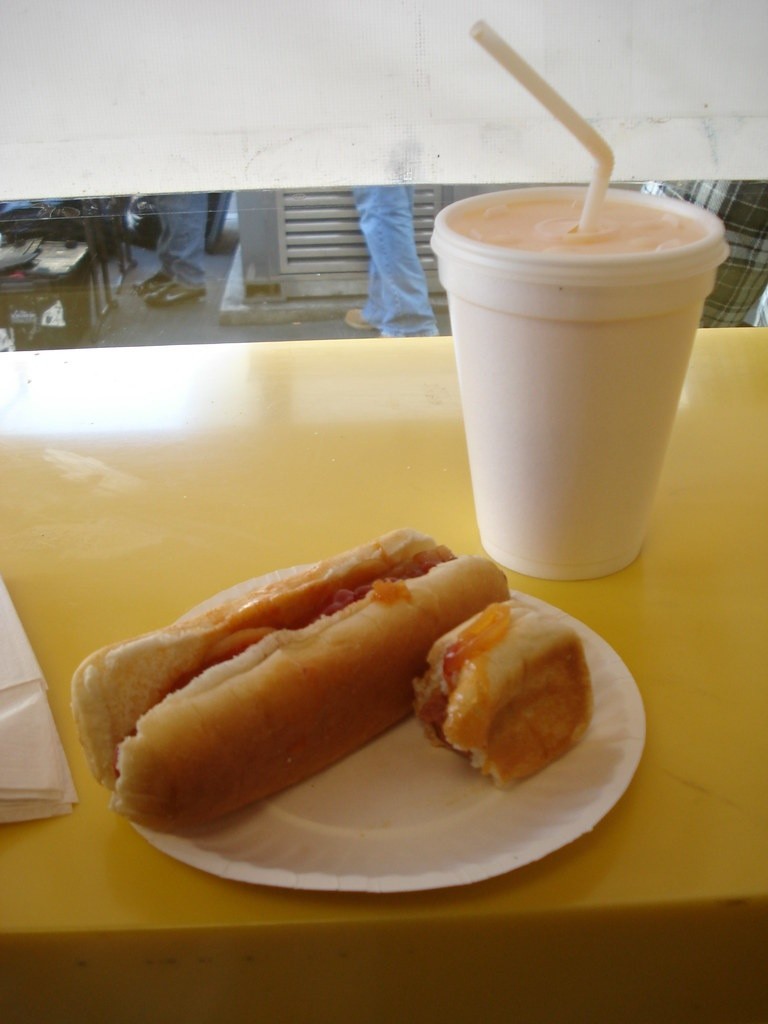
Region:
[135,273,171,297]
[346,308,381,331]
[143,278,207,306]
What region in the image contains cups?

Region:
[431,188,730,583]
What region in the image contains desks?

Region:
[0,330,768,1024]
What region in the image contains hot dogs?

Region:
[415,601,593,789]
[68,526,511,832]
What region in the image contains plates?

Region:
[118,567,644,890]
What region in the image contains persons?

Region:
[134,193,208,308]
[344,185,442,337]
